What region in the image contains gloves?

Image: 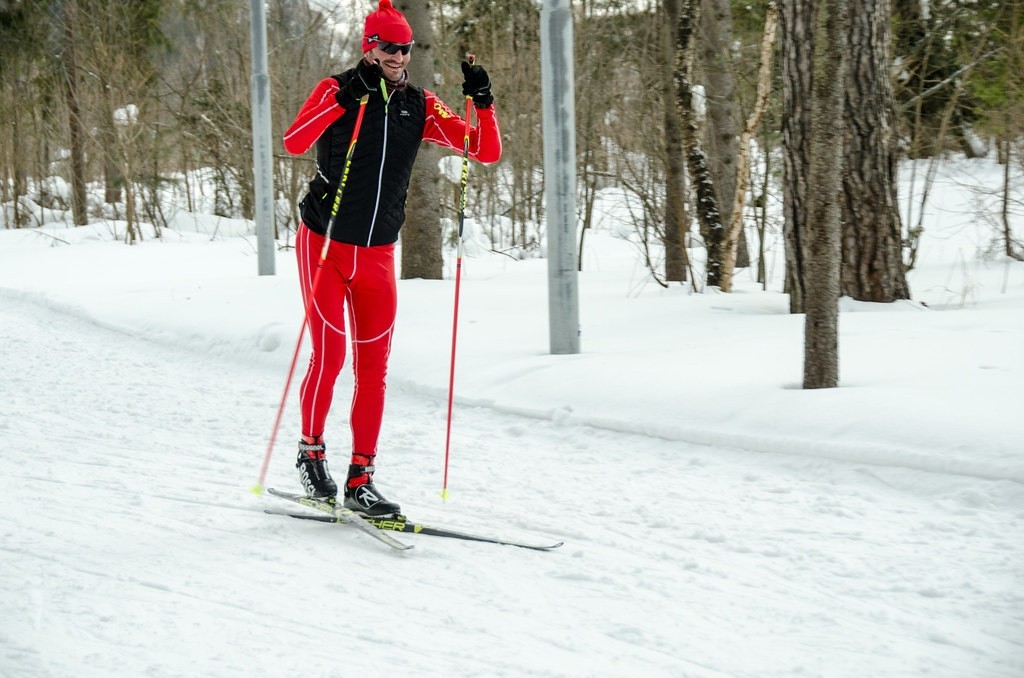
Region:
[461,61,493,109]
[346,59,383,102]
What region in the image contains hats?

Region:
[363,0,412,53]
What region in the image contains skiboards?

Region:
[263,487,565,552]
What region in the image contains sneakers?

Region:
[344,463,399,521]
[297,442,337,498]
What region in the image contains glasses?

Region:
[377,41,415,56]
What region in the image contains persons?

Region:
[283,0,503,519]
